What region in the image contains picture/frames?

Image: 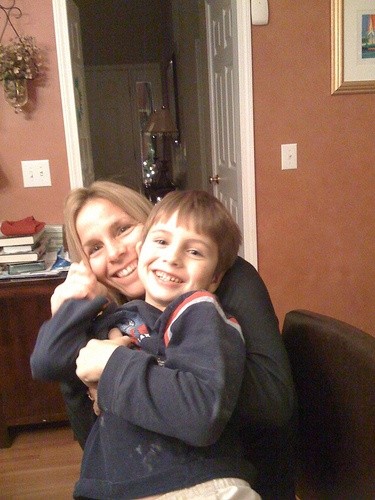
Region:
[330,0,375,95]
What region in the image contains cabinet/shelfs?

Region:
[0,276,70,448]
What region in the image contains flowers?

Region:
[0,34,39,102]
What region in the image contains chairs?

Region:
[281,309,374,500]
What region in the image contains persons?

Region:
[50,183,299,500]
[29,190,262,500]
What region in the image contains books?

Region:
[0,225,64,275]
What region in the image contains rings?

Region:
[88,394,94,401]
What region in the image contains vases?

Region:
[4,78,28,112]
